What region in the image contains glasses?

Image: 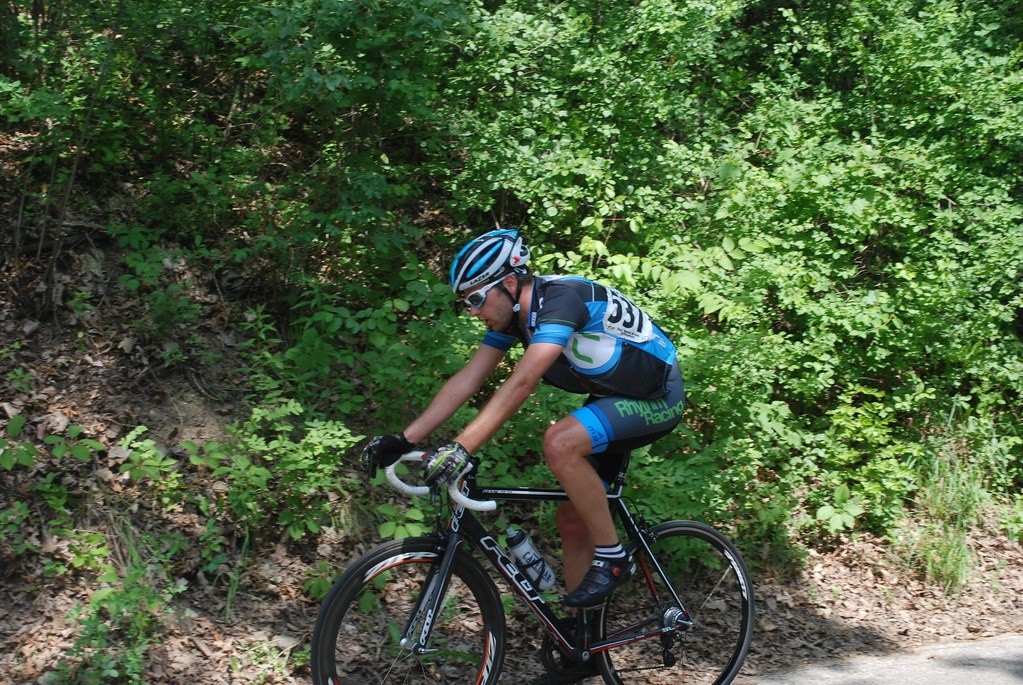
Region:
[448,270,518,308]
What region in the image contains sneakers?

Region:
[564,547,639,607]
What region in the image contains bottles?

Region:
[506,526,556,591]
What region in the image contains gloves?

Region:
[358,431,414,480]
[419,442,478,494]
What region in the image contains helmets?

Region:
[449,228,531,292]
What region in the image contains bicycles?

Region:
[311,440,756,685]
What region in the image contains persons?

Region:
[362,227,685,685]
